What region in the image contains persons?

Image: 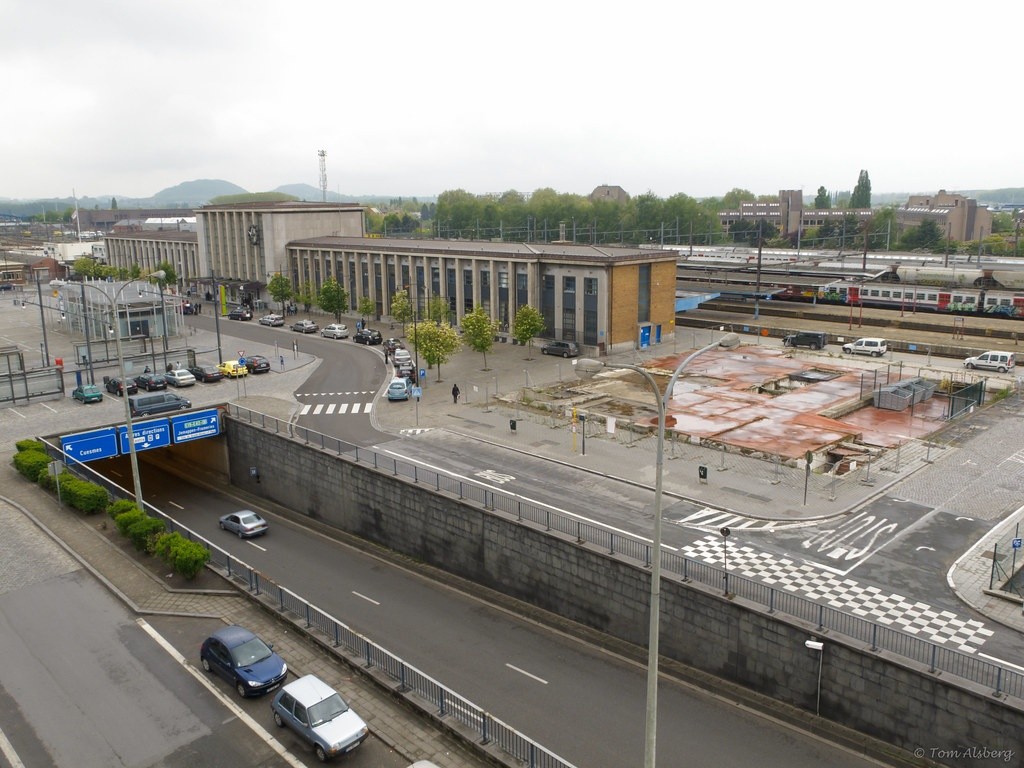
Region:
[176,361,182,370]
[167,363,173,371]
[360,318,367,330]
[269,309,274,315]
[451,383,460,404]
[144,365,152,373]
[286,303,298,316]
[280,355,285,371]
[194,301,204,314]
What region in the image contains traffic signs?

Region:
[117,417,170,454]
[58,427,118,466]
[169,409,220,444]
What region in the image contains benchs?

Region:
[494,335,506,342]
[28,391,46,396]
[0,395,11,401]
[513,338,526,346]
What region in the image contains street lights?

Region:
[44,247,61,278]
[396,277,426,327]
[266,264,297,319]
[48,269,166,515]
[571,333,740,768]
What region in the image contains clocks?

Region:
[248,225,260,245]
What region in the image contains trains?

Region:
[772,283,1024,317]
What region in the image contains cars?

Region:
[271,674,370,762]
[227,308,253,321]
[290,319,416,401]
[541,340,580,358]
[73,355,271,404]
[0,283,15,291]
[964,351,1016,373]
[258,314,284,327]
[220,509,269,538]
[842,338,888,358]
[200,625,288,698]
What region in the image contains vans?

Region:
[128,392,191,417]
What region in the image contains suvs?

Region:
[783,331,828,350]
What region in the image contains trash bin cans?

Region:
[55,358,62,366]
[892,381,925,406]
[872,387,912,410]
[904,378,937,400]
